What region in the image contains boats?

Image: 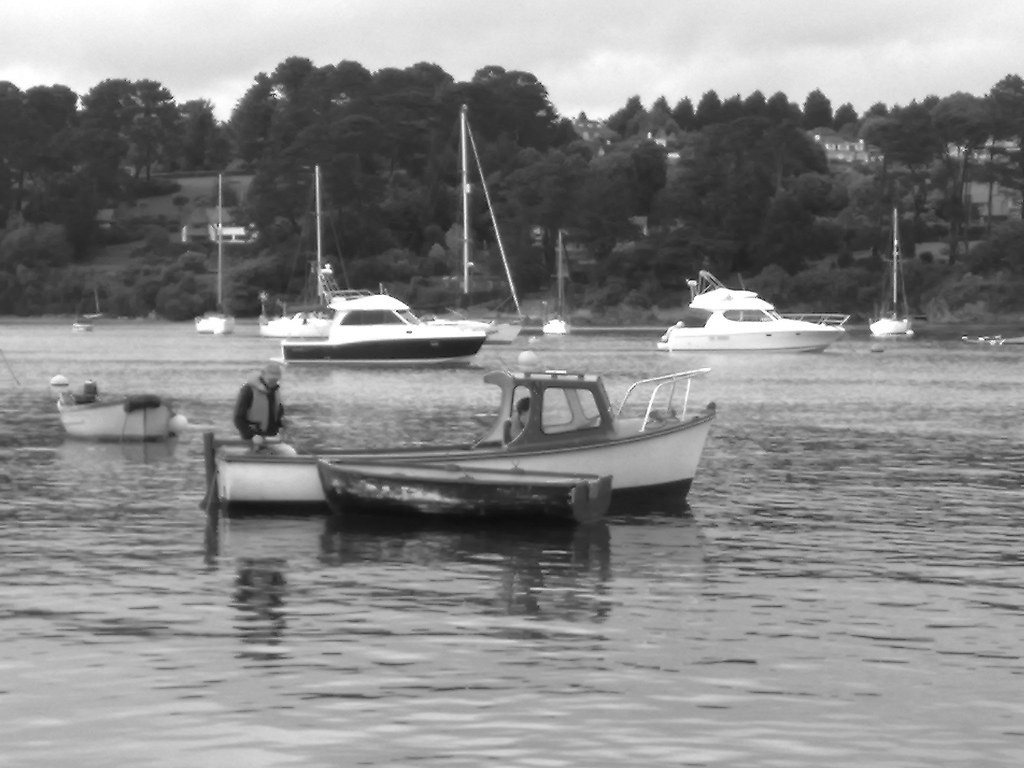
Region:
[72,321,97,332]
[212,364,718,518]
[316,455,615,530]
[83,313,104,321]
[51,373,186,441]
[654,270,851,357]
[282,295,487,359]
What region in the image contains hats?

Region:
[264,362,282,379]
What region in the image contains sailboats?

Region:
[867,205,919,338]
[195,178,239,335]
[258,105,527,360]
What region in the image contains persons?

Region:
[509,395,539,446]
[235,363,297,456]
